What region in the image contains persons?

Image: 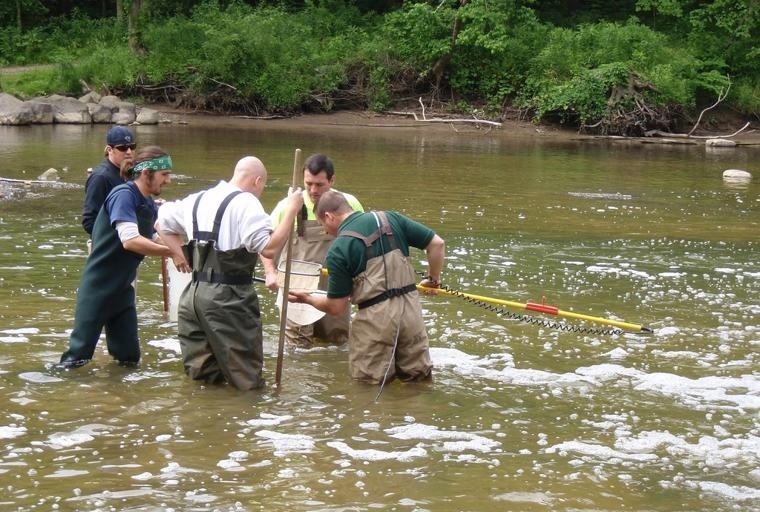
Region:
[46,145,172,377]
[156,156,304,389]
[287,191,444,383]
[259,154,365,348]
[80,125,137,258]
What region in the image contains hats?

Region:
[103,127,136,157]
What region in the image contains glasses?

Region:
[113,143,136,152]
